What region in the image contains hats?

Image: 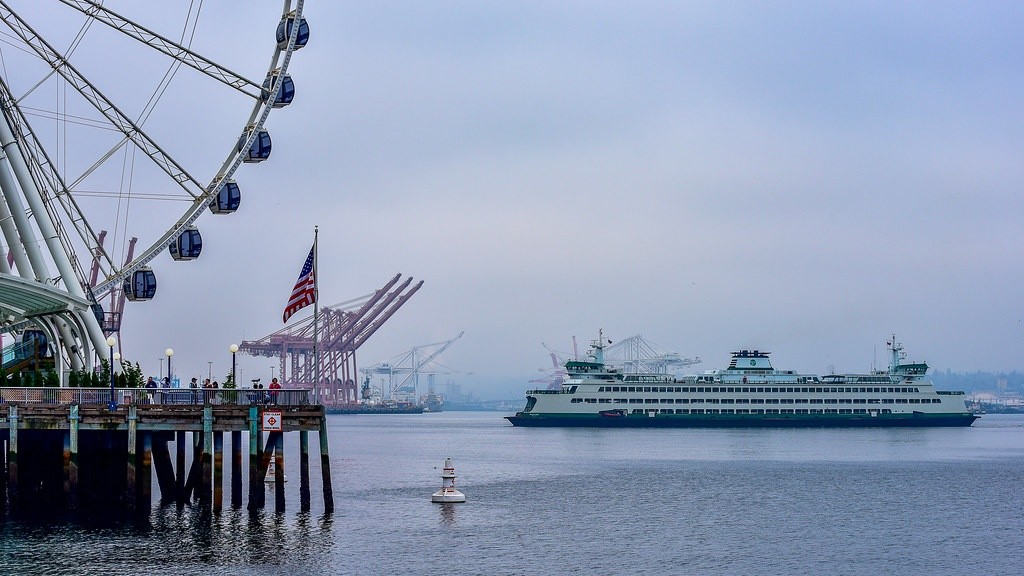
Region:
[192,378,197,380]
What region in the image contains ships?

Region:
[502,328,982,429]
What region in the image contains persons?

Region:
[250,377,281,405]
[189,377,199,405]
[202,379,219,405]
[161,377,171,404]
[145,377,157,404]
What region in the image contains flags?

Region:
[283,239,316,324]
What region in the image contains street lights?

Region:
[164,348,175,390]
[106,336,118,411]
[229,343,239,389]
